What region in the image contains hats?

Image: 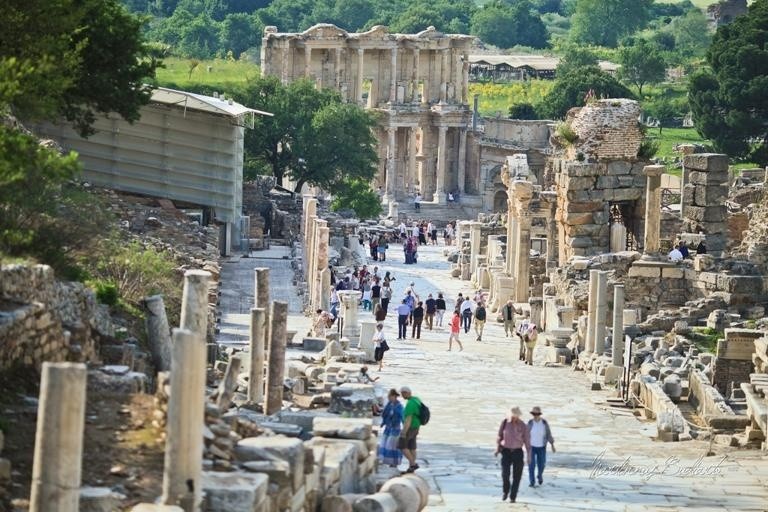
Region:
[511,406,522,415]
[530,407,542,414]
[389,387,409,396]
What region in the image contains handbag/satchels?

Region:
[380,340,389,352]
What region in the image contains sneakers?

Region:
[407,464,418,473]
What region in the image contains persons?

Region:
[526,405,557,487]
[493,406,533,504]
[356,367,380,385]
[667,245,683,263]
[396,385,422,476]
[375,389,405,468]
[312,216,540,373]
[413,192,423,213]
[679,241,689,259]
[446,191,454,209]
[697,240,708,254]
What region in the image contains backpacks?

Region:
[413,402,429,425]
